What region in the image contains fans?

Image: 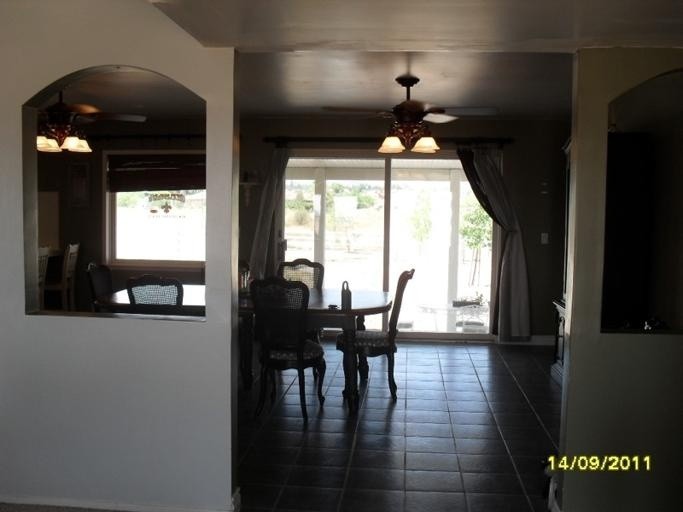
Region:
[321,73,498,125]
[47,86,150,128]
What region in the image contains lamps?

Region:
[35,86,94,154]
[375,74,440,157]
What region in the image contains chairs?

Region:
[38,245,53,304]
[48,244,78,309]
[334,268,415,404]
[242,278,331,422]
[83,262,115,311]
[274,257,326,344]
[123,273,185,318]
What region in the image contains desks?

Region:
[105,280,392,408]
